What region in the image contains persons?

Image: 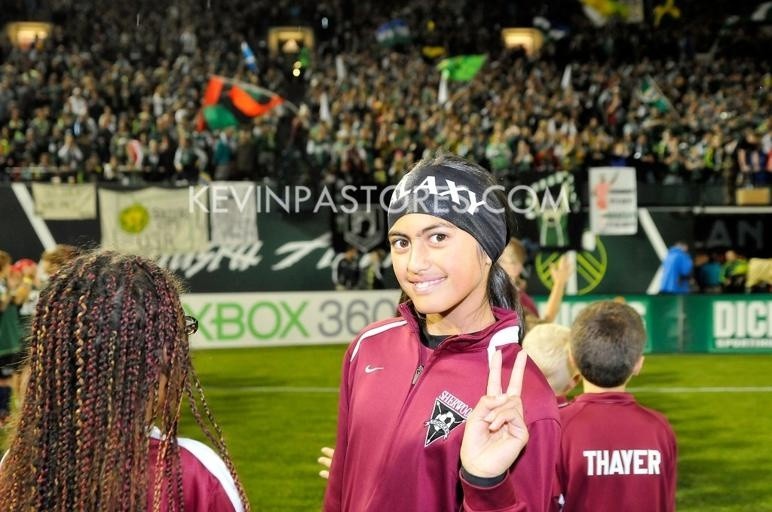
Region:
[317,150,567,512]
[0,0,772,208]
[495,237,581,410]
[1,242,85,316]
[657,235,771,292]
[0,248,253,511]
[360,248,387,291]
[331,245,361,291]
[558,303,675,511]
[316,445,335,482]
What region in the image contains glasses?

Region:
[183,317,198,340]
